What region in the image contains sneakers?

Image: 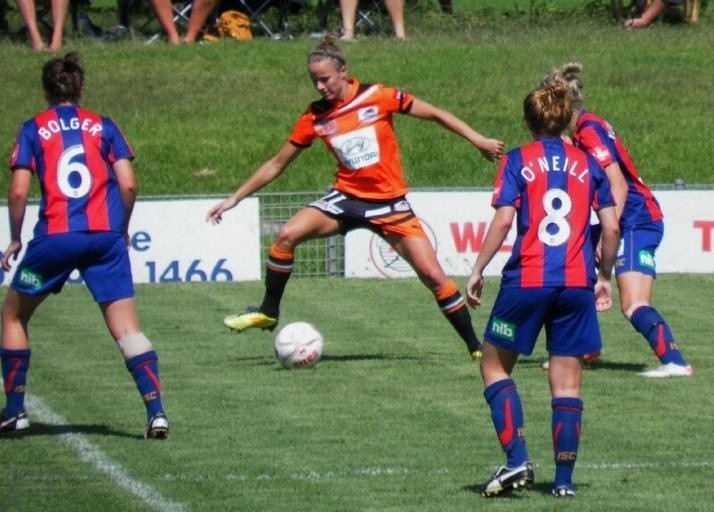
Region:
[552,485,574,498]
[481,461,533,497]
[144,412,168,438]
[640,361,692,377]
[0,408,30,433]
[224,307,278,332]
[543,351,600,370]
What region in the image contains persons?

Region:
[624,0,668,28]
[0,52,169,438]
[339,0,405,41]
[147,0,218,45]
[16,0,70,51]
[204,35,505,362]
[466,77,621,498]
[541,62,695,379]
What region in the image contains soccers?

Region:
[274,322,323,369]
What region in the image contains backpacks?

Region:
[215,10,252,40]
